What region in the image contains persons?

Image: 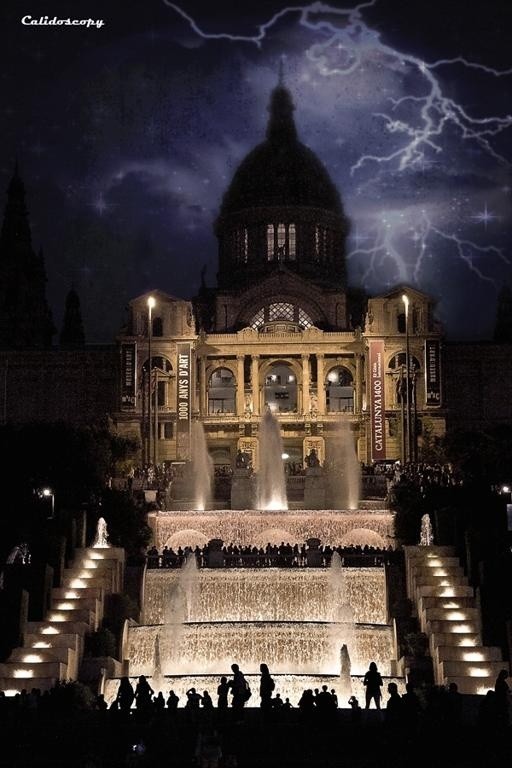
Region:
[226,663,247,709]
[281,698,292,709]
[259,663,273,708]
[347,696,358,709]
[201,690,213,708]
[1,680,109,713]
[166,689,179,710]
[493,668,510,694]
[115,676,135,711]
[153,691,165,710]
[184,687,202,708]
[386,682,401,711]
[103,460,172,512]
[145,542,394,568]
[135,675,155,705]
[217,676,230,708]
[362,662,383,711]
[401,683,417,699]
[298,685,339,710]
[272,693,283,709]
[360,460,463,493]
[212,466,233,481]
[283,461,305,477]
